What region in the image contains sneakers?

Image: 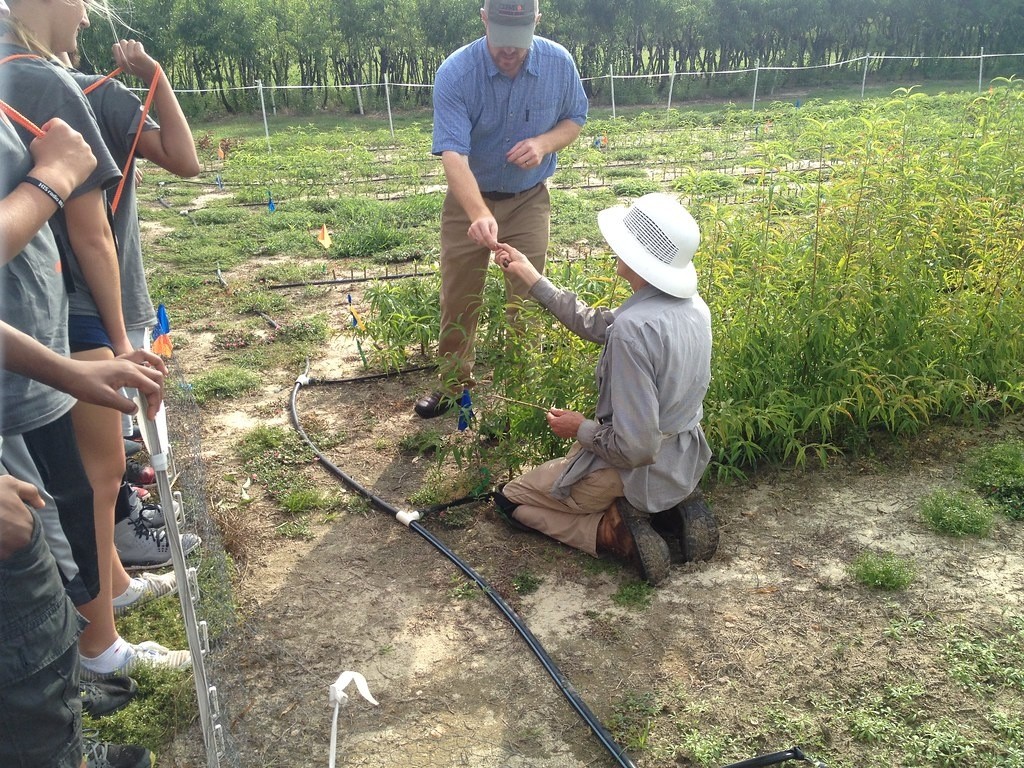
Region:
[114,479,178,527]
[74,641,191,686]
[108,564,188,620]
[70,669,136,722]
[109,494,203,569]
[78,737,157,768]
[414,384,455,417]
[122,456,156,498]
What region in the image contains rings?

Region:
[525,160,529,167]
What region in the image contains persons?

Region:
[2,1,198,768]
[414,0,589,420]
[483,193,721,586]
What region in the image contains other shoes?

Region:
[670,480,724,567]
[604,492,673,590]
[123,438,142,455]
[123,424,143,440]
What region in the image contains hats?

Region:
[596,197,702,303]
[480,0,542,51]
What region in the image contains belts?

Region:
[475,187,540,203]
